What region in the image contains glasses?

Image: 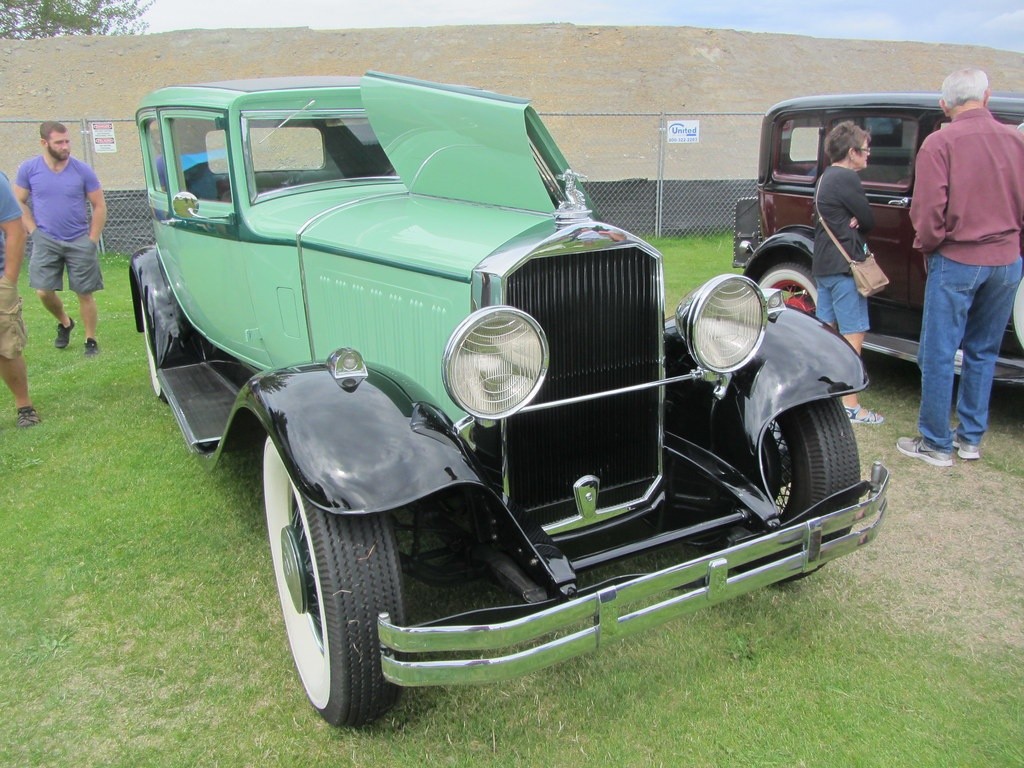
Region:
[856,147,870,155]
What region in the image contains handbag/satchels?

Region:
[850,253,889,298]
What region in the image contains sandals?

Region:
[844,403,883,425]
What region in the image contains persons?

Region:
[810,118,889,422]
[895,65,1024,468]
[0,170,42,427]
[13,121,108,355]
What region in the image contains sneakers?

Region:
[84,337,98,357]
[952,427,980,459]
[17,405,39,429]
[897,436,953,466]
[55,317,75,348]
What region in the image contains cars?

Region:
[735,92,1024,393]
[125,70,895,729]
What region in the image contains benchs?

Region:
[859,146,913,183]
[223,170,345,201]
